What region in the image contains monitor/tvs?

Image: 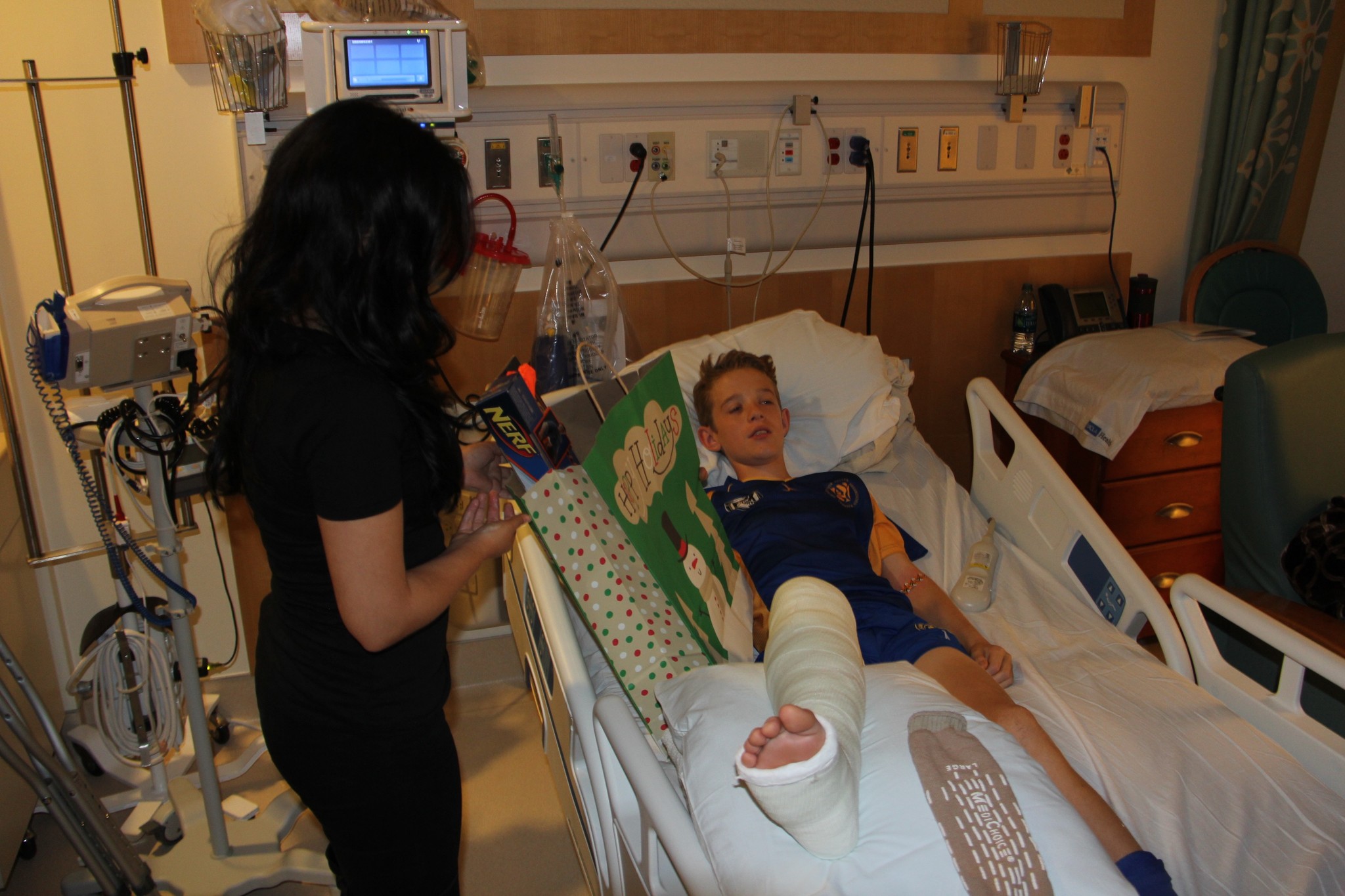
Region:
[336,26,441,106]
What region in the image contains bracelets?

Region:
[899,573,928,596]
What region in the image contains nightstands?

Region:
[1019,400,1226,647]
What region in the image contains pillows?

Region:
[1009,320,1273,463]
[610,307,917,488]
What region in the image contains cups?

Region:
[456,190,531,344]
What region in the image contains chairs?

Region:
[1193,332,1345,744]
[1179,236,1327,350]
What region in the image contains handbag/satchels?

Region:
[496,335,757,762]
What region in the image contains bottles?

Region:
[1012,284,1035,356]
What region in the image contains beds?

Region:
[496,306,1344,895]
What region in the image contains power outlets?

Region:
[1088,125,1111,168]
[1051,123,1074,169]
[844,125,867,174]
[821,127,844,175]
[623,132,649,182]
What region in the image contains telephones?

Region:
[1038,283,1131,349]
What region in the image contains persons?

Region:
[210,99,528,896]
[691,348,1180,896]
